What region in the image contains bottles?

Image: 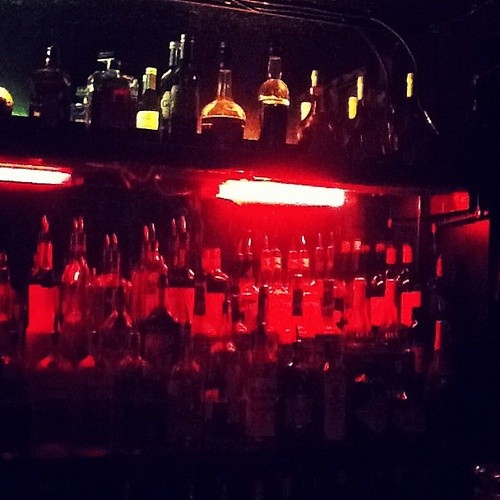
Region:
[1,20,436,160]
[2,202,446,470]
